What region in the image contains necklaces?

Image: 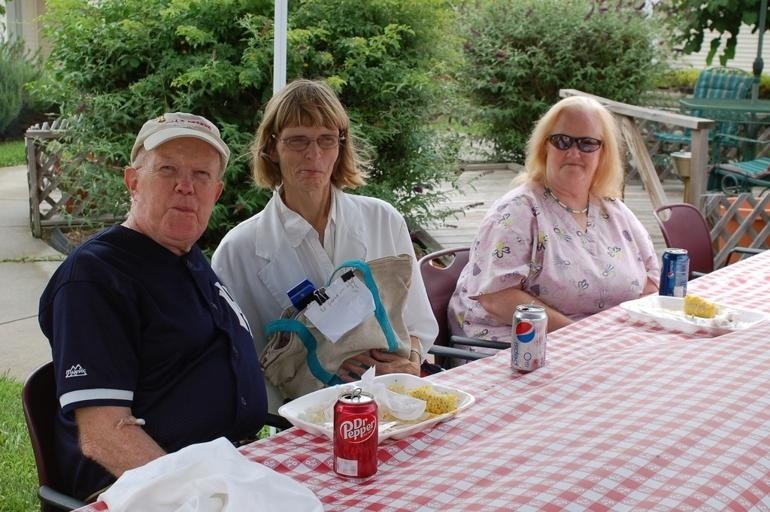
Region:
[544,184,588,214]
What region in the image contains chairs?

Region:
[624,71,752,187]
[714,153,770,195]
[429,341,492,365]
[419,246,517,348]
[21,361,292,511]
[654,202,766,277]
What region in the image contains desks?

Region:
[678,90,769,160]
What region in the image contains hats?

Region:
[130,111,231,179]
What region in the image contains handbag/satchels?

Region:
[258,250,416,396]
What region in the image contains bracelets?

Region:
[411,349,422,364]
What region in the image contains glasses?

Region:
[547,134,603,153]
[271,135,345,152]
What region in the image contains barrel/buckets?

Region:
[717,196,769,270]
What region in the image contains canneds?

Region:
[660,248,690,298]
[333,388,379,479]
[511,303,548,372]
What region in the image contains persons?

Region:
[39,111,267,503]
[447,94,663,371]
[211,77,440,437]
